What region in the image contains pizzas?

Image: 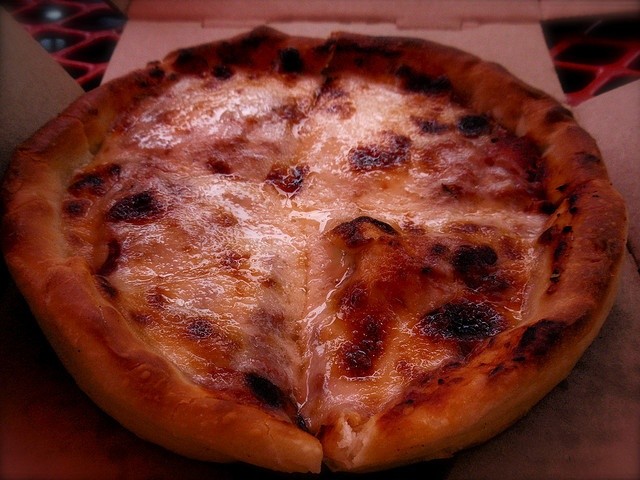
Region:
[0,22,628,472]
